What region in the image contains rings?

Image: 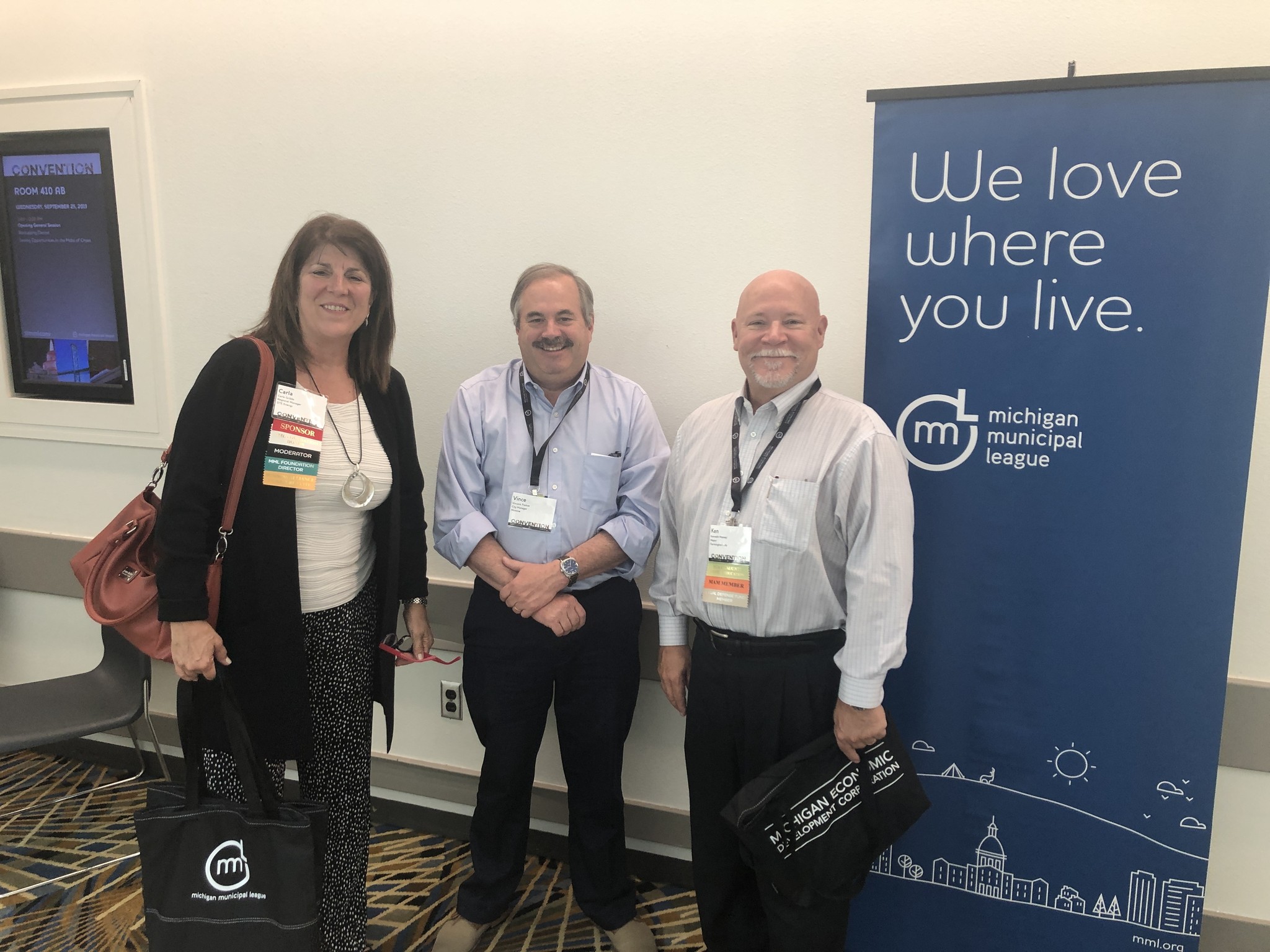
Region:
[514,605,521,611]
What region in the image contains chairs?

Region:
[0,624,170,899]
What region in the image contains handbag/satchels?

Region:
[720,718,930,906]
[70,336,277,663]
[133,669,335,952]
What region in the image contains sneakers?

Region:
[431,905,512,952]
[588,918,657,952]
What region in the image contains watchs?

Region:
[556,556,579,587]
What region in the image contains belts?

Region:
[693,616,847,656]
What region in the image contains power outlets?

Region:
[440,680,463,720]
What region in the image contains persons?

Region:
[154,214,433,952]
[434,265,672,952]
[646,270,915,952]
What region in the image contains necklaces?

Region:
[301,359,375,508]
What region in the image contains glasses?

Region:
[378,634,462,665]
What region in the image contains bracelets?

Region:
[849,705,867,711]
[401,597,428,608]
